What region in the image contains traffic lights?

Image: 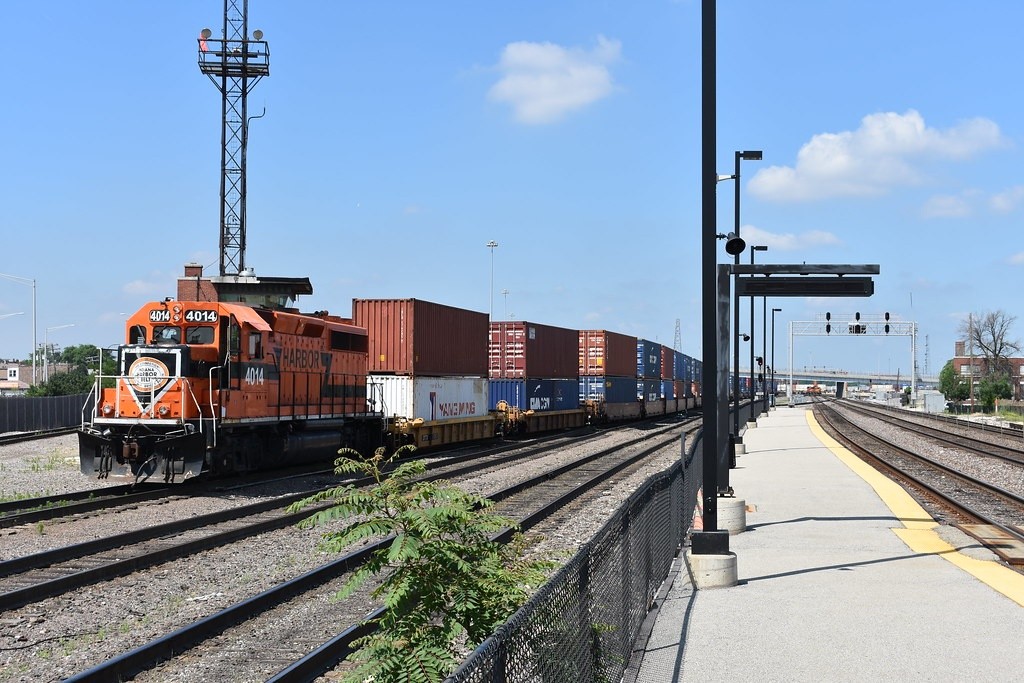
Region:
[758,374,762,382]
[758,357,762,365]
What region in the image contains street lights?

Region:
[733,148,764,454]
[768,307,782,412]
[44,322,75,386]
[487,239,499,321]
[500,287,509,320]
[745,245,769,429]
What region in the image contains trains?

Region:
[78,297,756,492]
